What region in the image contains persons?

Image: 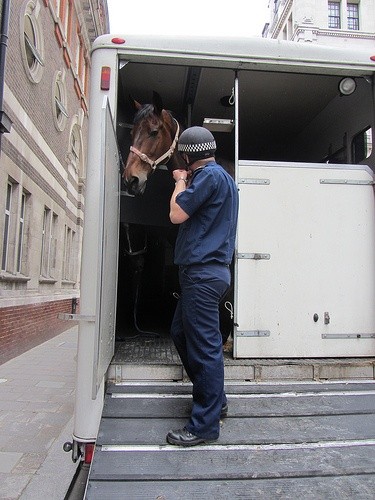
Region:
[165,126,240,448]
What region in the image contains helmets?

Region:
[178,126,216,165]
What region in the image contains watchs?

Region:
[175,177,187,183]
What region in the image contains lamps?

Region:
[338,75,358,97]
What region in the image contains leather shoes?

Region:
[168,426,219,446]
[221,404,229,417]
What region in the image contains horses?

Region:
[121,98,235,347]
